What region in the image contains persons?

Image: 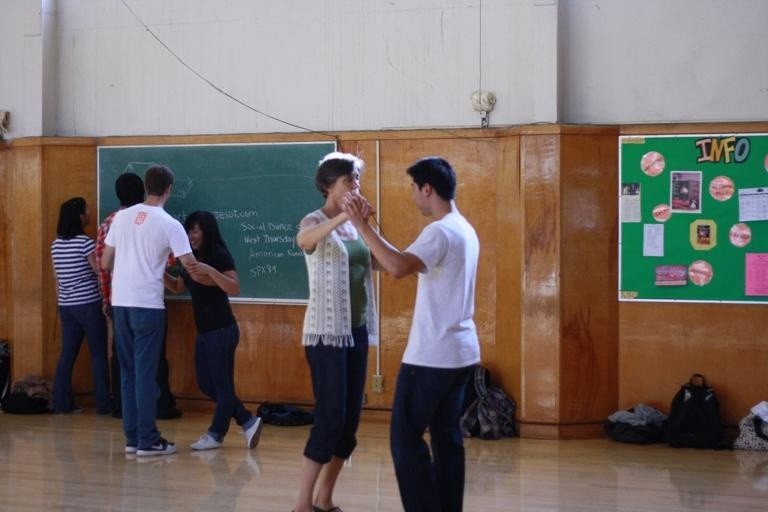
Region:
[46,417,263,511]
[164,210,263,450]
[339,156,483,511]
[50,196,114,416]
[291,151,388,512]
[96,172,183,419]
[100,165,218,456]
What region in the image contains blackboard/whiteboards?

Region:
[619,132,768,305]
[96,141,338,306]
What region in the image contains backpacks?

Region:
[257,401,313,427]
[1,391,48,414]
[605,406,667,443]
[0,338,10,402]
[667,373,723,449]
[463,365,517,438]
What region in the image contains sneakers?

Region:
[125,437,176,457]
[95,404,122,417]
[55,404,86,414]
[189,433,223,449]
[245,416,263,449]
[157,403,182,420]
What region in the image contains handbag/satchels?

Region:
[11,375,55,412]
[731,410,768,451]
